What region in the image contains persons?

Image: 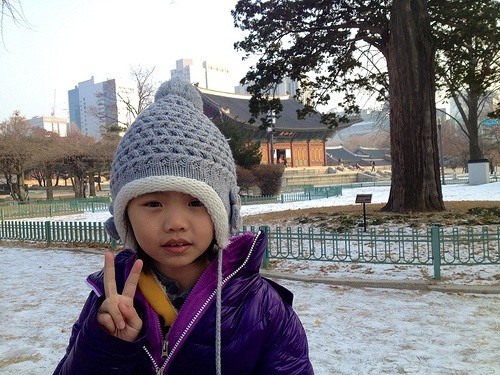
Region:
[338,158,360,169]
[371,160,375,171]
[53,77,314,375]
[463,158,468,173]
[283,158,287,167]
[490,162,496,174]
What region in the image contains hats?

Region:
[109,76,242,251]
